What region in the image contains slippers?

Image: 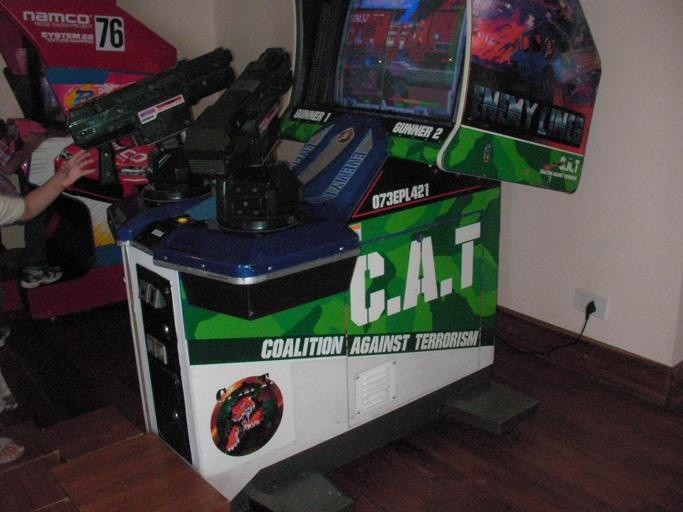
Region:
[0,433,25,464]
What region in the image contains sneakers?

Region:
[18,264,64,290]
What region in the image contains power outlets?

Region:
[573,290,608,320]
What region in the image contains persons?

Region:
[0,148,98,467]
[0,129,64,292]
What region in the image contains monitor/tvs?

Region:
[301,1,467,122]
